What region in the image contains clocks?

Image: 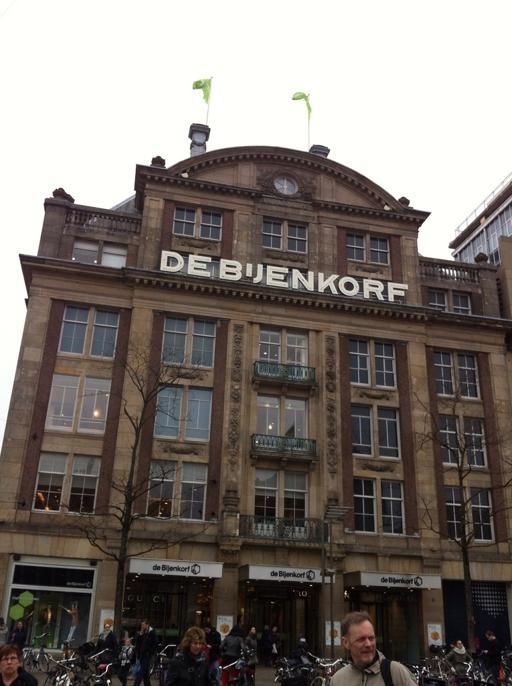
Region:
[274,175,298,196]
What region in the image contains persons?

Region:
[164,626,212,686]
[132,618,157,686]
[442,639,473,674]
[96,618,128,686]
[9,620,26,654]
[203,622,281,686]
[0,644,39,686]
[482,630,503,686]
[328,610,419,686]
[0,617,11,650]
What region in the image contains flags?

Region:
[291,91,312,119]
[192,78,211,103]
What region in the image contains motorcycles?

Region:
[273,638,315,686]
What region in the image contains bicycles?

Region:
[307,647,512,686]
[22,632,256,685]
